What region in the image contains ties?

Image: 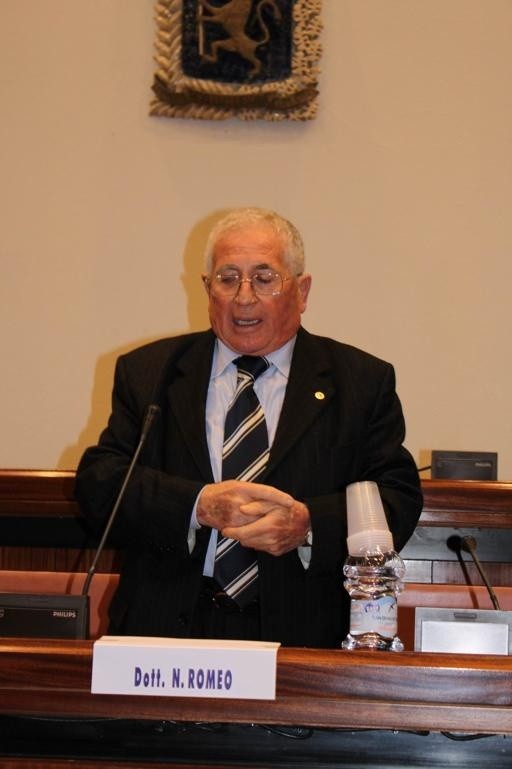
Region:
[214,355,270,609]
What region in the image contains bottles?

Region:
[340,544,406,652]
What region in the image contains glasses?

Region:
[215,269,296,296]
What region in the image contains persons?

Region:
[71,207,423,650]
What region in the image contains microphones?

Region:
[461,535,501,612]
[82,403,161,595]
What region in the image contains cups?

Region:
[346,479,396,557]
